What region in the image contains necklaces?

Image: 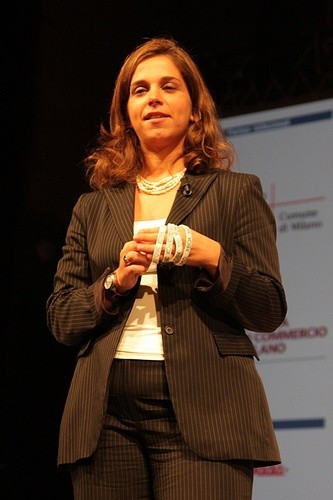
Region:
[136,167,187,195]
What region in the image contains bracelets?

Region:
[152,223,192,266]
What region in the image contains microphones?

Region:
[181,183,193,197]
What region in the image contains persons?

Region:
[47,38,288,500]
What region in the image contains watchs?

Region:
[104,271,129,300]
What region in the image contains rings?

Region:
[123,255,131,266]
